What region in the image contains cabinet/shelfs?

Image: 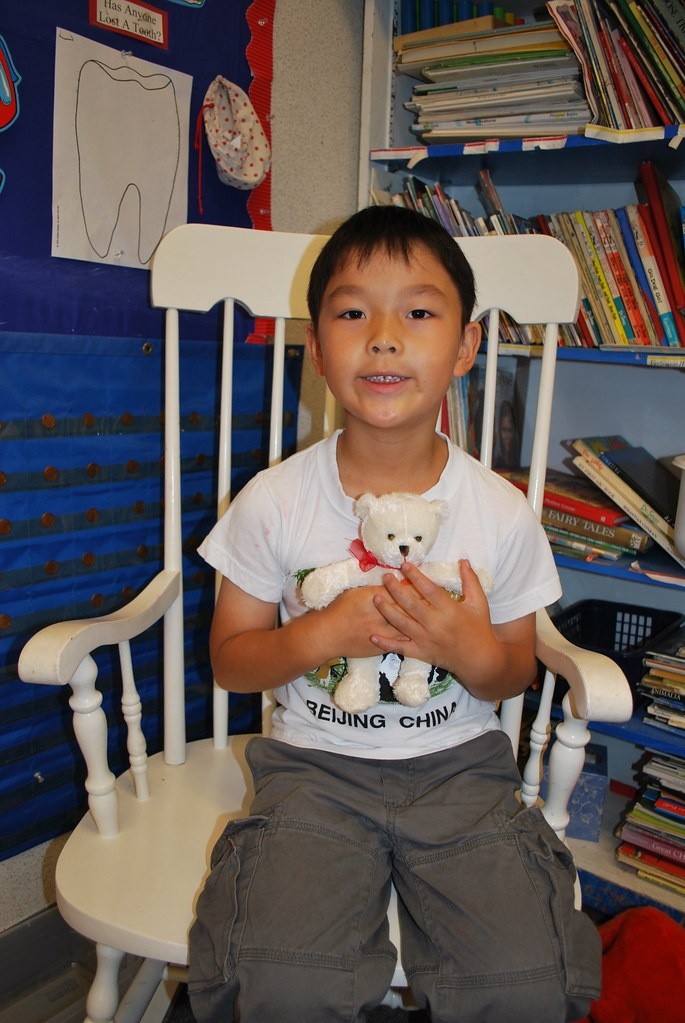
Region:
[359,0,685,915]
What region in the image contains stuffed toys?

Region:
[301,492,492,714]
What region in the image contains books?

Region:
[641,621,685,737]
[369,162,685,348]
[446,364,685,570]
[393,0,685,136]
[614,747,685,895]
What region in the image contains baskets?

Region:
[540,599,685,713]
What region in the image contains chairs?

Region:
[19,225,633,1023]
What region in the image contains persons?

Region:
[494,401,520,468]
[197,206,602,1023]
